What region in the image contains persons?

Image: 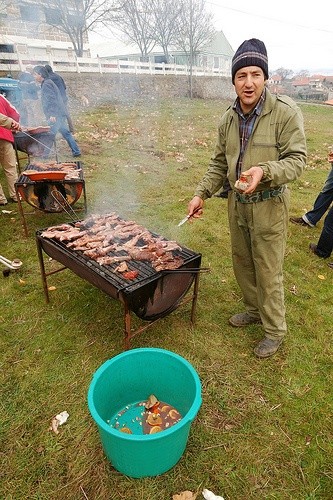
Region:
[291,152,333,256]
[20,66,38,121]
[187,39,308,358]
[0,114,21,131]
[33,65,82,159]
[46,65,74,135]
[1,95,25,205]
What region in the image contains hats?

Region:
[231,38,269,85]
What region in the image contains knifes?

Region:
[178,206,202,227]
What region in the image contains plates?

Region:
[22,171,68,181]
[28,127,50,134]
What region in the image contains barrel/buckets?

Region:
[86,348,202,478]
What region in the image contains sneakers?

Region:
[254,336,284,358]
[228,311,260,328]
[289,215,312,229]
[309,242,318,255]
